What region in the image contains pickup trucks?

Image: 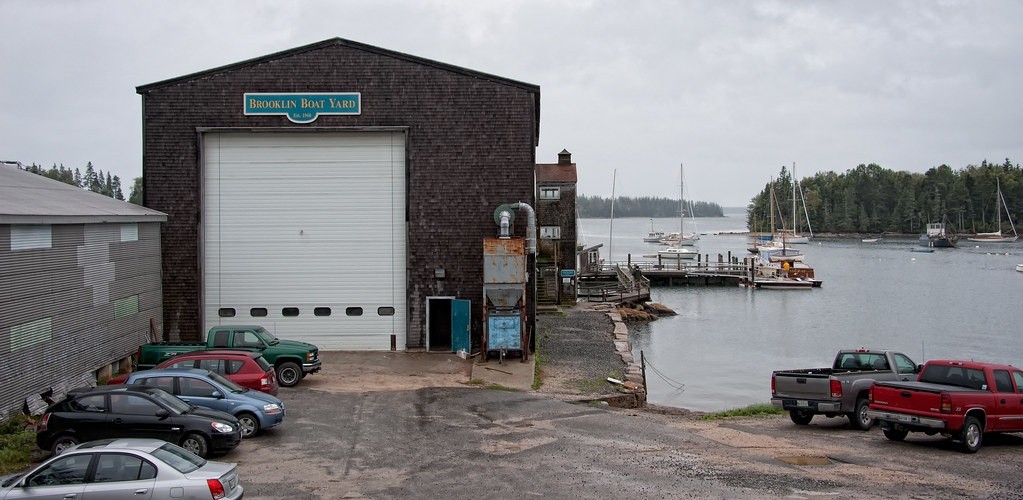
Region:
[867,358,1023,452]
[133,324,323,388]
[770,345,927,430]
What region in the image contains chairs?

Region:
[203,361,214,370]
[843,358,860,367]
[996,378,1004,390]
[950,374,964,384]
[874,359,887,369]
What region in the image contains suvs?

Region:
[105,350,280,397]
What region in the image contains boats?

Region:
[643,218,670,241]
[747,206,770,254]
[918,213,959,247]
[752,175,823,287]
[910,247,935,252]
[862,238,884,242]
[659,233,695,247]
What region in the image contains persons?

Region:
[781,261,789,278]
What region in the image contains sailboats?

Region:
[657,163,700,259]
[577,208,588,250]
[772,161,814,245]
[747,221,766,246]
[965,175,1018,242]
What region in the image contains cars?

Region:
[1,435,245,500]
[36,385,243,459]
[124,370,287,438]
